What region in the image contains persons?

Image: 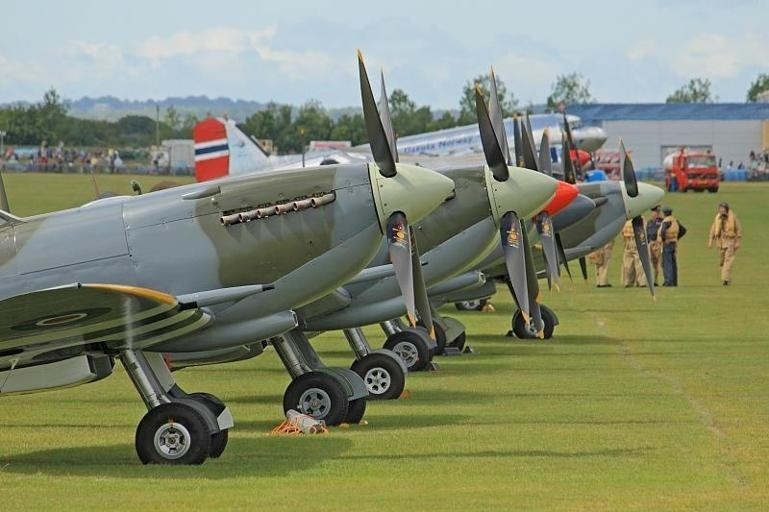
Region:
[708,203,743,286]
[657,206,686,287]
[588,238,614,287]
[647,204,667,286]
[6,141,123,176]
[718,145,768,181]
[620,213,647,288]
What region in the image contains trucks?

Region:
[665,145,720,192]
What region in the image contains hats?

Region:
[650,205,661,212]
[662,207,672,213]
[720,202,728,207]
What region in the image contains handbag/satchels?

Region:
[678,225,687,240]
